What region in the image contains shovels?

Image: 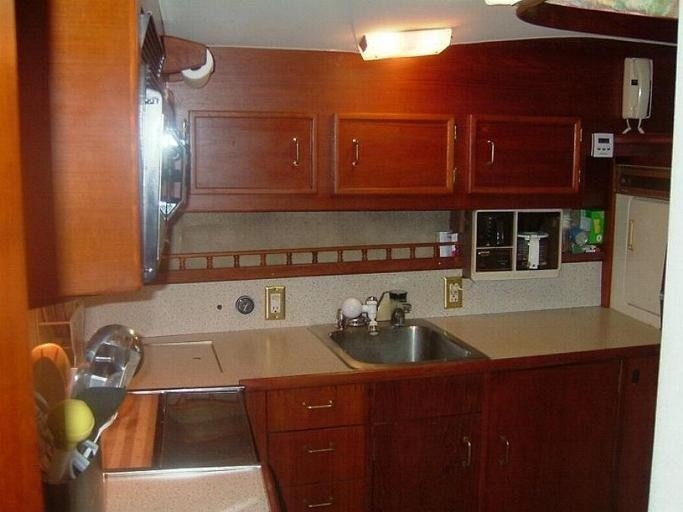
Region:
[78,386,126,458]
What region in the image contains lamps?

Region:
[356,23,453,61]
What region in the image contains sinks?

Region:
[306,318,489,370]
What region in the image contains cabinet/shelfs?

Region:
[622,345,661,512]
[608,189,670,330]
[17,0,170,307]
[374,349,624,512]
[324,51,462,210]
[457,209,563,280]
[462,40,587,208]
[188,48,323,212]
[239,372,373,512]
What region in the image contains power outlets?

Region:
[445,276,462,308]
[264,285,286,321]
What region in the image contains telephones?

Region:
[623,58,654,118]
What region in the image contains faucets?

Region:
[342,296,381,336]
